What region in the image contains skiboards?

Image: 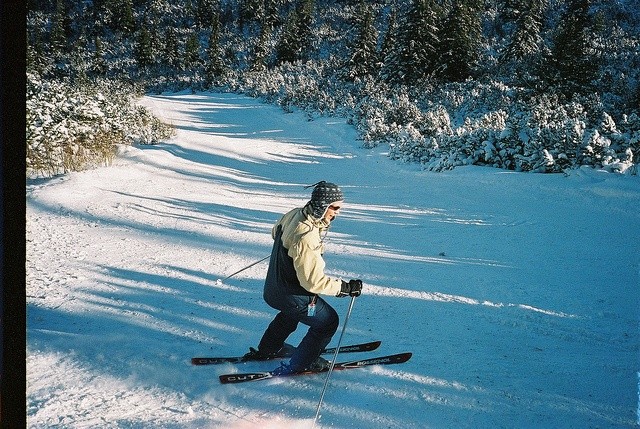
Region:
[192,340,412,384]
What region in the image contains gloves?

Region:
[336,278,362,297]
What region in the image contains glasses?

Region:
[330,205,340,211]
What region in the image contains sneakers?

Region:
[259,341,297,356]
[290,356,331,370]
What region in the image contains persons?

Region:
[258,179,363,371]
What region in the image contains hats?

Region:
[304,180,344,226]
